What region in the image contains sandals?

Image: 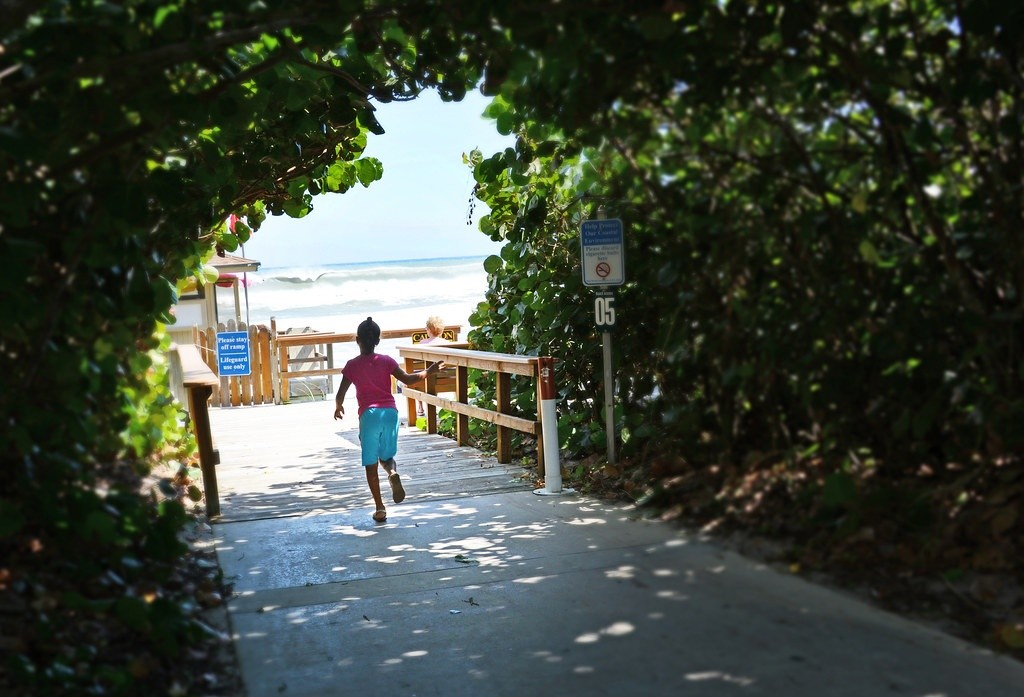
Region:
[372,510,387,522]
[388,471,405,503]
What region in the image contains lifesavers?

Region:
[216,273,253,288]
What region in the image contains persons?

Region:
[333,316,446,522]
